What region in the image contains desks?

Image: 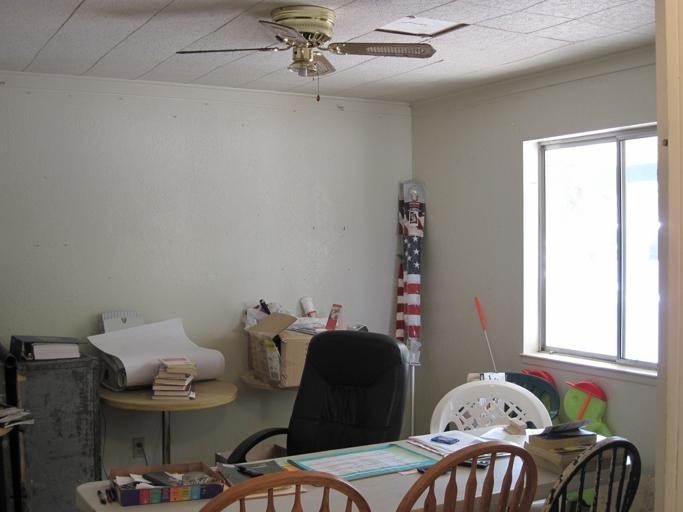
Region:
[71,420,636,512]
[94,375,237,465]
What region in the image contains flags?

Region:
[394,182,427,343]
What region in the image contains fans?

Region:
[169,5,438,79]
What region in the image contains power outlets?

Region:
[132,436,145,460]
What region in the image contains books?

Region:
[215,459,291,494]
[405,414,627,476]
[149,357,197,400]
[0,401,35,429]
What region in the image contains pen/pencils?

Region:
[234,464,264,477]
[97,487,118,504]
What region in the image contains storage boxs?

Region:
[246,312,345,389]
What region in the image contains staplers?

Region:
[504,418,527,436]
